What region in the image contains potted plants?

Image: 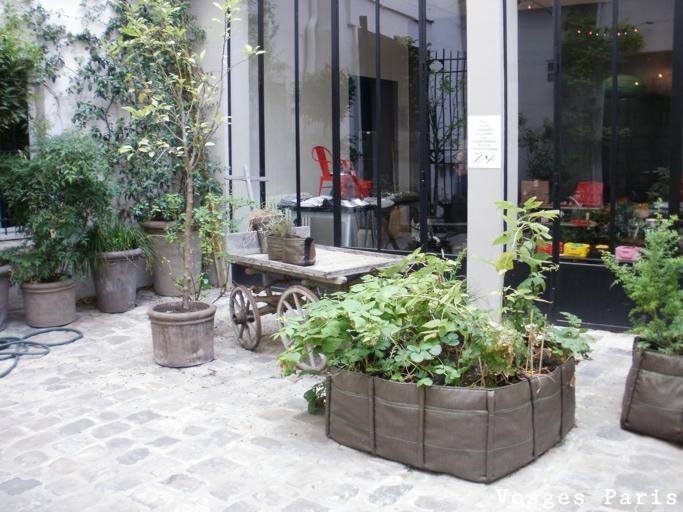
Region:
[596,199,683,447]
[270,193,594,484]
[278,222,316,264]
[265,214,294,260]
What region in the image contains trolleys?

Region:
[218,225,426,375]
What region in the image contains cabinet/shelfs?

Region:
[519,203,604,230]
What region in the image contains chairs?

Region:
[577,181,604,206]
[310,145,370,194]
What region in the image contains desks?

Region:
[277,189,420,252]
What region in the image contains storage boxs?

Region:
[520,179,550,203]
